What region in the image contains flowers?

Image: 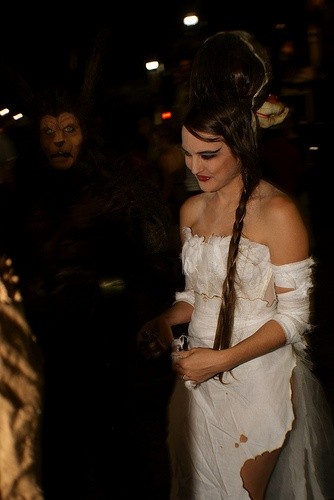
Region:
[256,94,290,128]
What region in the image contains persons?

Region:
[136,98,312,500]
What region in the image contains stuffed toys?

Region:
[0,31,327,500]
